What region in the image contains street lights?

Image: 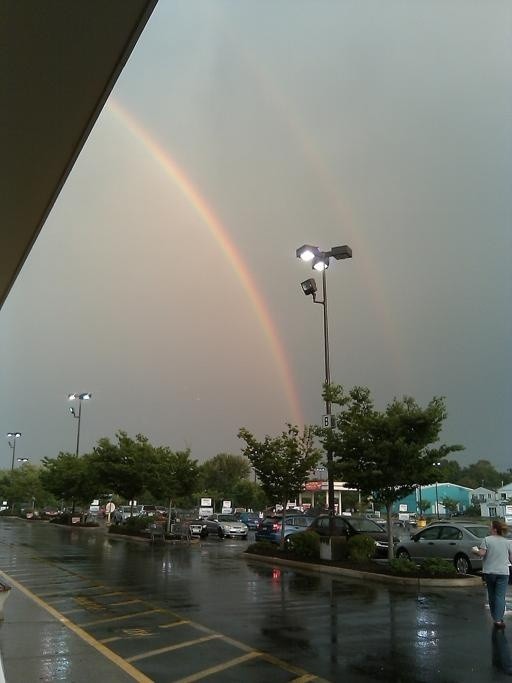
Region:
[294,242,353,561]
[67,391,91,514]
[7,431,21,469]
[17,457,28,464]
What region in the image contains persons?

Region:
[469,520,511,631]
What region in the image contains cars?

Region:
[391,522,512,574]
[110,503,259,539]
[21,504,89,520]
[285,514,400,561]
[256,515,320,551]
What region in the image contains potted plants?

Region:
[347,533,377,566]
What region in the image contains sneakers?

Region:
[493,618,505,628]
